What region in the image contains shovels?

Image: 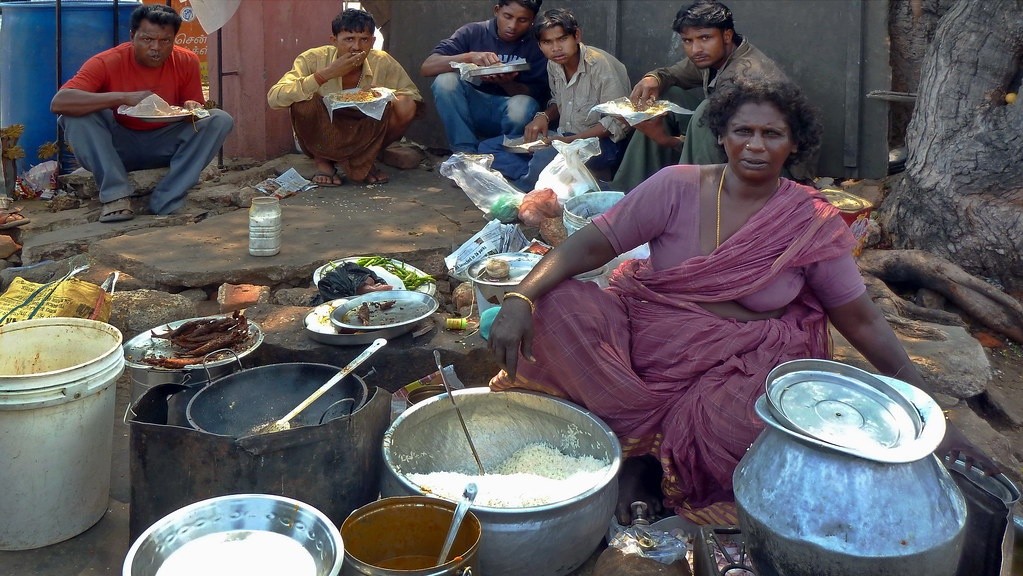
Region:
[261,338,387,433]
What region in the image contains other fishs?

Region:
[149,311,248,370]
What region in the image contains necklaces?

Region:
[717,162,782,250]
[356,73,362,89]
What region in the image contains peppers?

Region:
[329,255,438,292]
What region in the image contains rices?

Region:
[336,91,374,102]
[388,424,612,510]
[625,97,666,114]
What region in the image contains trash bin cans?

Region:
[0,0,143,177]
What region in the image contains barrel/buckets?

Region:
[0,316,125,551]
[562,191,626,236]
[340,496,481,576]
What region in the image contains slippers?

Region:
[312,168,340,187]
[100,197,135,221]
[364,162,389,183]
[170,208,207,222]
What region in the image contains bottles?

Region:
[446,318,468,330]
[249,197,281,256]
[733,373,966,576]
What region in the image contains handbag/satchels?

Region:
[0,261,119,323]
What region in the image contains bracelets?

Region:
[502,292,536,314]
[534,111,550,123]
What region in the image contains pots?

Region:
[186,347,368,438]
[379,387,623,576]
[129,350,254,408]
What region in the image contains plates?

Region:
[501,143,548,154]
[302,296,420,345]
[330,87,390,104]
[468,63,531,76]
[129,106,190,122]
[596,98,668,116]
[332,290,439,328]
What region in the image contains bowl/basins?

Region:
[121,493,344,576]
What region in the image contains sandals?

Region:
[0,210,30,229]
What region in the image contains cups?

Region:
[405,385,459,410]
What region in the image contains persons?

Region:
[421,0,548,188]
[50,4,233,223]
[486,75,1003,523]
[477,9,631,194]
[266,8,423,187]
[0,137,31,230]
[599,0,821,197]
